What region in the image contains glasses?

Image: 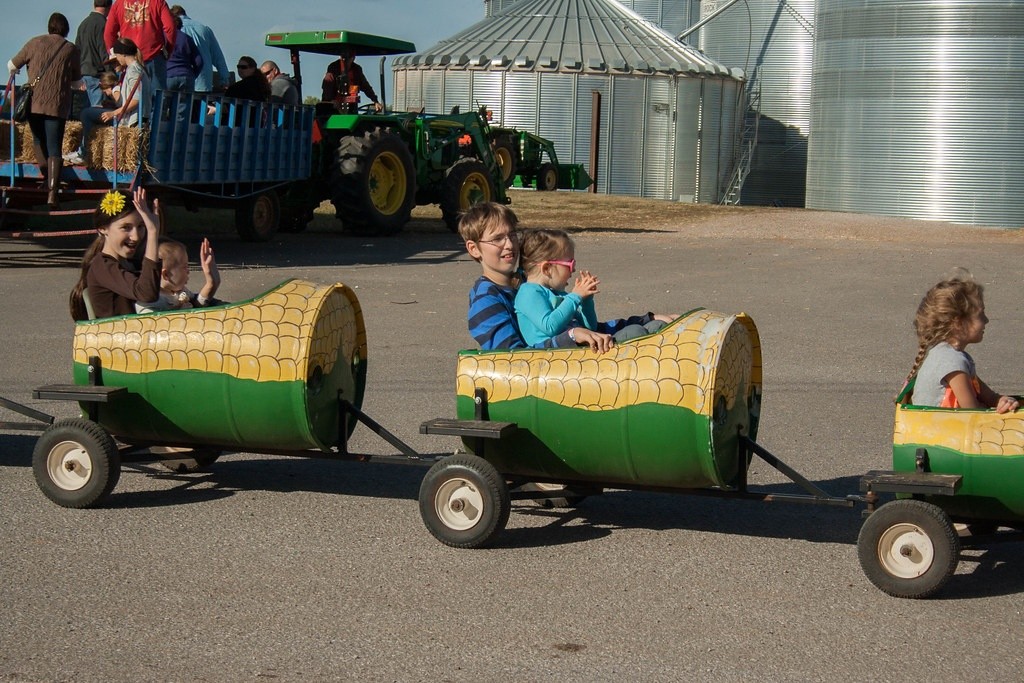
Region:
[535,259,576,273]
[474,229,525,246]
[263,66,276,76]
[237,64,253,70]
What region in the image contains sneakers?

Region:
[60,152,88,166]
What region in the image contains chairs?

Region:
[316,101,346,153]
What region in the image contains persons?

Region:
[62,38,152,165]
[161,15,203,121]
[207,56,272,127]
[459,200,680,355]
[894,279,1021,414]
[78,0,114,106]
[134,238,195,313]
[8,12,81,210]
[171,5,230,123]
[104,0,178,119]
[513,229,667,346]
[261,60,301,128]
[322,48,383,114]
[69,184,221,320]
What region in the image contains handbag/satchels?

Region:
[14,83,33,122]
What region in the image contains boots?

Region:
[47,157,64,211]
[32,145,50,192]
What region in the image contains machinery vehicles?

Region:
[0,30,511,243]
[492,125,596,193]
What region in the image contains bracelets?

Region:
[568,328,575,341]
[198,295,210,303]
